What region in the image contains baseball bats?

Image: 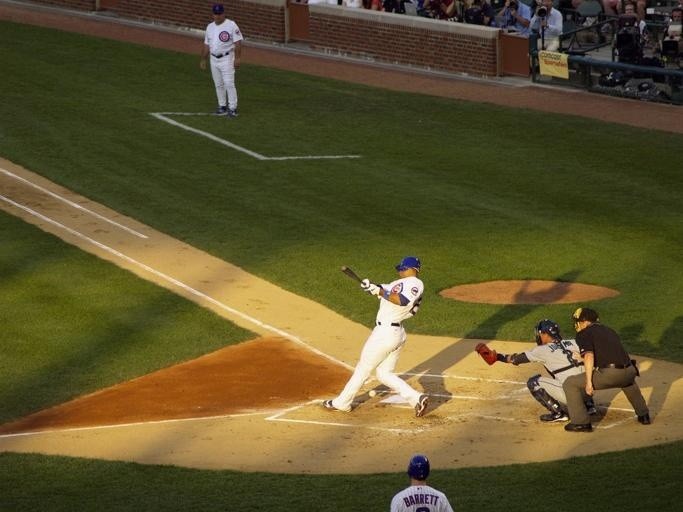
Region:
[342,266,380,298]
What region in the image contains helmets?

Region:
[407,455,430,481]
[535,318,563,346]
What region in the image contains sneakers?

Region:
[228,109,238,117]
[323,399,352,413]
[216,107,228,116]
[539,412,569,423]
[414,393,429,418]
[583,398,597,415]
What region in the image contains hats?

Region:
[212,5,224,14]
[571,307,599,324]
[395,256,422,273]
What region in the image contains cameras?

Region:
[537,5,548,17]
[507,0,517,10]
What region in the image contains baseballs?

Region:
[369,390,376,397]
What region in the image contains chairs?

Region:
[517,1,683,72]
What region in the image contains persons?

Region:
[308,1,505,27]
[475,318,596,423]
[388,455,453,511]
[317,256,431,418]
[561,307,650,432]
[604,0,683,59]
[196,4,245,117]
[499,1,583,51]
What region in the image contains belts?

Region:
[375,322,400,326]
[598,362,631,370]
[210,52,229,58]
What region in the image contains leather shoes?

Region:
[637,414,651,425]
[564,422,592,433]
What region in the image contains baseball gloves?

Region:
[475,343,497,365]
